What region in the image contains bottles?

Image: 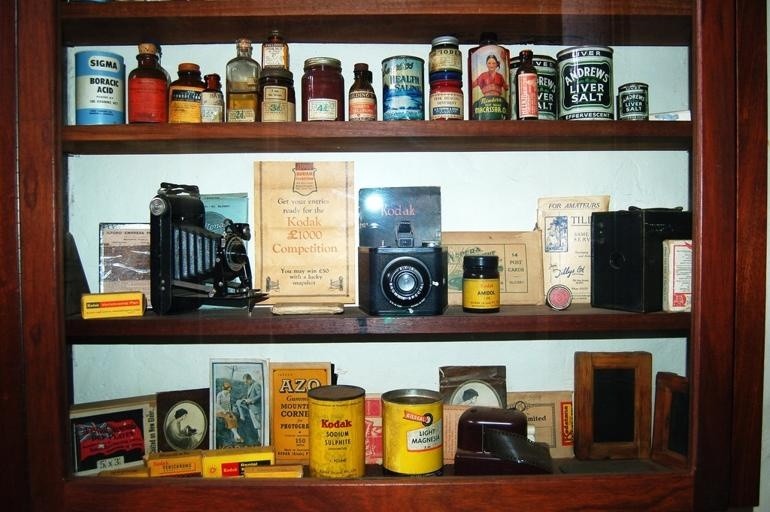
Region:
[461,254,500,312]
[127,28,378,124]
[514,49,539,120]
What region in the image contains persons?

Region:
[243,373,261,447]
[217,382,245,445]
[166,408,198,449]
[455,388,479,406]
[471,54,509,98]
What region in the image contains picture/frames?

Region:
[573,351,654,462]
[651,370,690,469]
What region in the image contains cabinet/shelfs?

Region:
[0,0,769,510]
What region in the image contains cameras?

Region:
[150,182,269,316]
[359,241,447,315]
[591,205,692,313]
